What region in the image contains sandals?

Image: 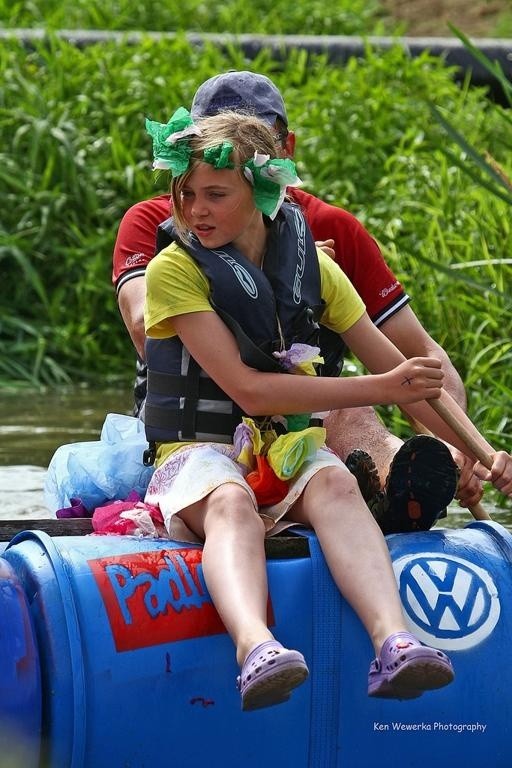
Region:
[238,638,309,712]
[368,633,458,698]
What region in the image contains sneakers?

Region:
[385,432,459,531]
[346,446,389,531]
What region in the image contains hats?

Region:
[192,69,289,129]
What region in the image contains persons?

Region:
[105,68,487,534]
[145,112,511,716]
